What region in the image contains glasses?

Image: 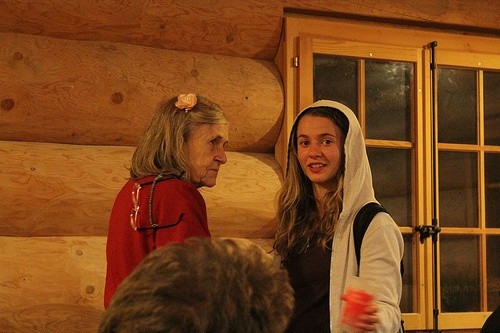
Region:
[130,169,186,231]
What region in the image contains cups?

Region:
[339,287,373,333]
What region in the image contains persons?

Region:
[273,99,405,333]
[96,238,294,333]
[103,93,230,311]
